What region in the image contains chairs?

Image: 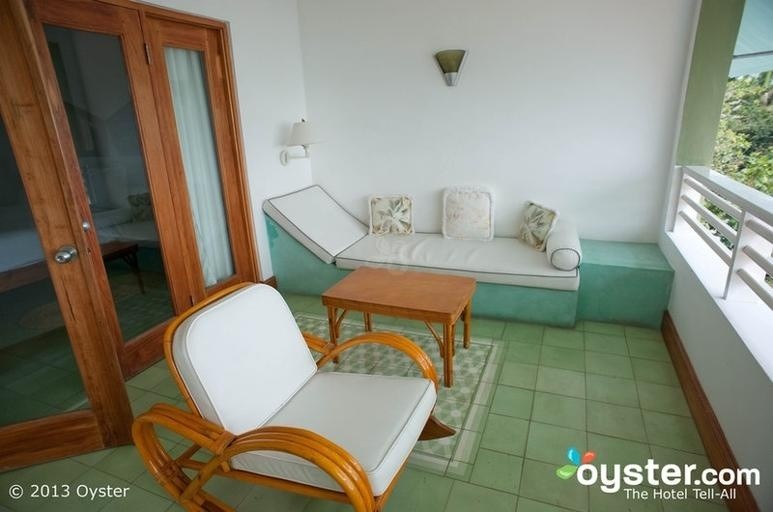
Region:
[133,280,456,512]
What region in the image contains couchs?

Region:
[89,204,166,273]
[262,185,582,330]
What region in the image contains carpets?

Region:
[290,311,504,477]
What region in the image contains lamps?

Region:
[280,119,319,166]
[434,50,468,85]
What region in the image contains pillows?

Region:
[92,206,131,230]
[545,220,581,270]
[368,194,415,237]
[517,201,558,251]
[443,187,494,241]
[128,194,154,222]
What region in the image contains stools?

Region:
[578,237,675,329]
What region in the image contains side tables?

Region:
[322,265,476,387]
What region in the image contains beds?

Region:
[0,206,133,295]
